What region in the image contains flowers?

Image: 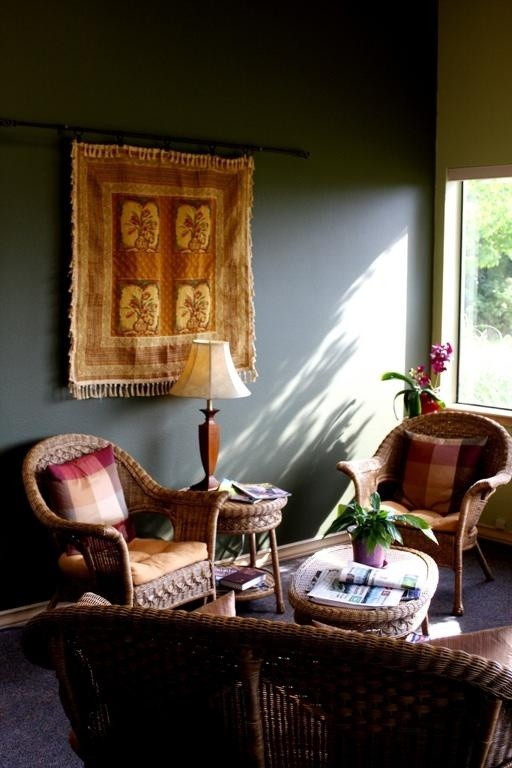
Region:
[382,341,453,419]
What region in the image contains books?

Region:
[214,568,238,588]
[219,567,267,592]
[216,477,262,505]
[232,482,292,501]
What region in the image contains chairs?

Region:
[22,433,232,610]
[335,410,512,616]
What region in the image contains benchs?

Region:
[19,591,512,768]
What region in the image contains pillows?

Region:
[390,427,489,517]
[46,444,136,557]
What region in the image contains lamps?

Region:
[166,338,253,493]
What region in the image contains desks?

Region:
[288,540,440,638]
[177,485,289,615]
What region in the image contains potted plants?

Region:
[322,492,441,571]
